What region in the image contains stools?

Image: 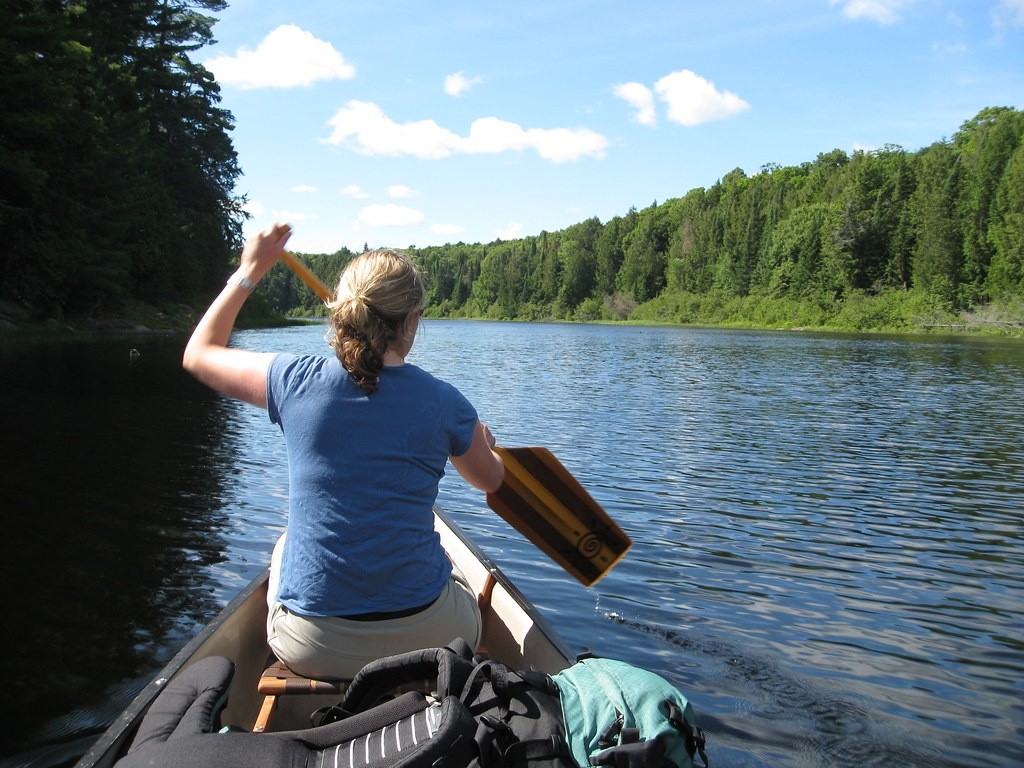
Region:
[256,650,434,694]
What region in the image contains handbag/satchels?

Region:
[550,656,710,768]
[461,659,563,768]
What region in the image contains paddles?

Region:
[279,247,634,589]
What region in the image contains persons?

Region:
[183,223,504,681]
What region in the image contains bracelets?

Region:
[227,275,255,293]
